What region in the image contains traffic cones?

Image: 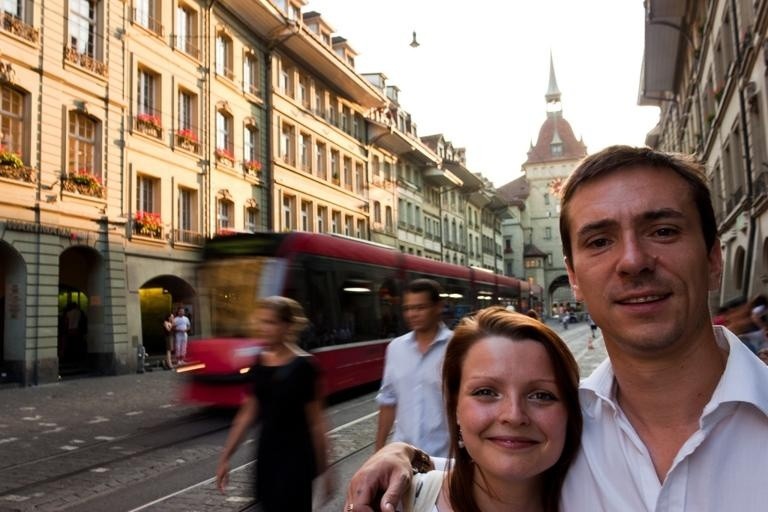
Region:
[585,339,595,350]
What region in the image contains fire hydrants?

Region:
[136,342,149,376]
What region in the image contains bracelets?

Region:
[416,448,432,475]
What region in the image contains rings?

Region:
[345,503,353,512]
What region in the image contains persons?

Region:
[515,292,597,340]
[339,142,766,512]
[160,310,178,369]
[722,294,758,335]
[172,306,192,366]
[385,305,585,512]
[373,277,457,462]
[214,294,339,512]
[711,305,729,329]
[737,292,767,366]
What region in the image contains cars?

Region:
[551,301,585,325]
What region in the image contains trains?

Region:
[165,230,551,414]
